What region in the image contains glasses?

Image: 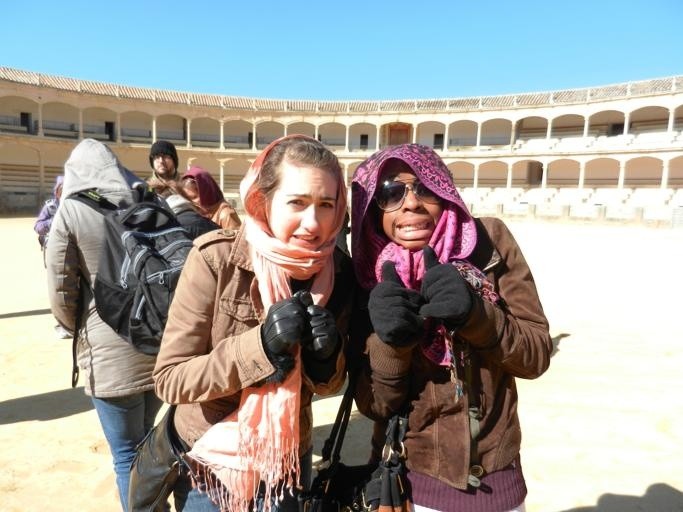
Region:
[370,178,445,211]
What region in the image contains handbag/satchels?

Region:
[127,404,191,512]
[298,368,418,512]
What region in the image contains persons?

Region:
[140,139,187,204]
[176,162,242,232]
[342,141,552,512]
[32,173,73,342]
[146,175,223,238]
[45,136,191,512]
[152,130,359,511]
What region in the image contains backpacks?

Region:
[62,184,194,358]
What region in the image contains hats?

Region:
[148,141,179,170]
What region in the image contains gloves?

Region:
[415,244,476,329]
[259,289,312,365]
[363,259,424,349]
[299,292,341,363]
